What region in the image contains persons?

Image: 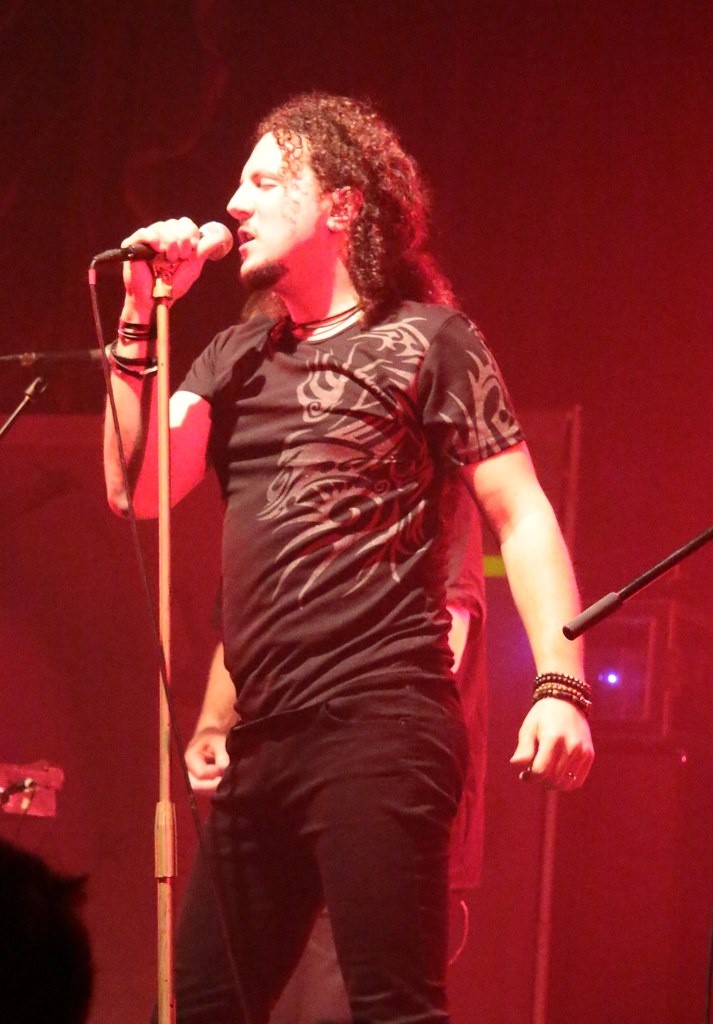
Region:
[104,90,595,1024]
[180,472,490,1024]
[0,842,96,1024]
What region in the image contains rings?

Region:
[567,772,576,780]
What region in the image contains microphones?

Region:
[91,221,234,264]
[10,343,115,368]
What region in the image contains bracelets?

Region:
[531,672,593,716]
[106,318,159,378]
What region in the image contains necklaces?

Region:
[279,304,362,334]
